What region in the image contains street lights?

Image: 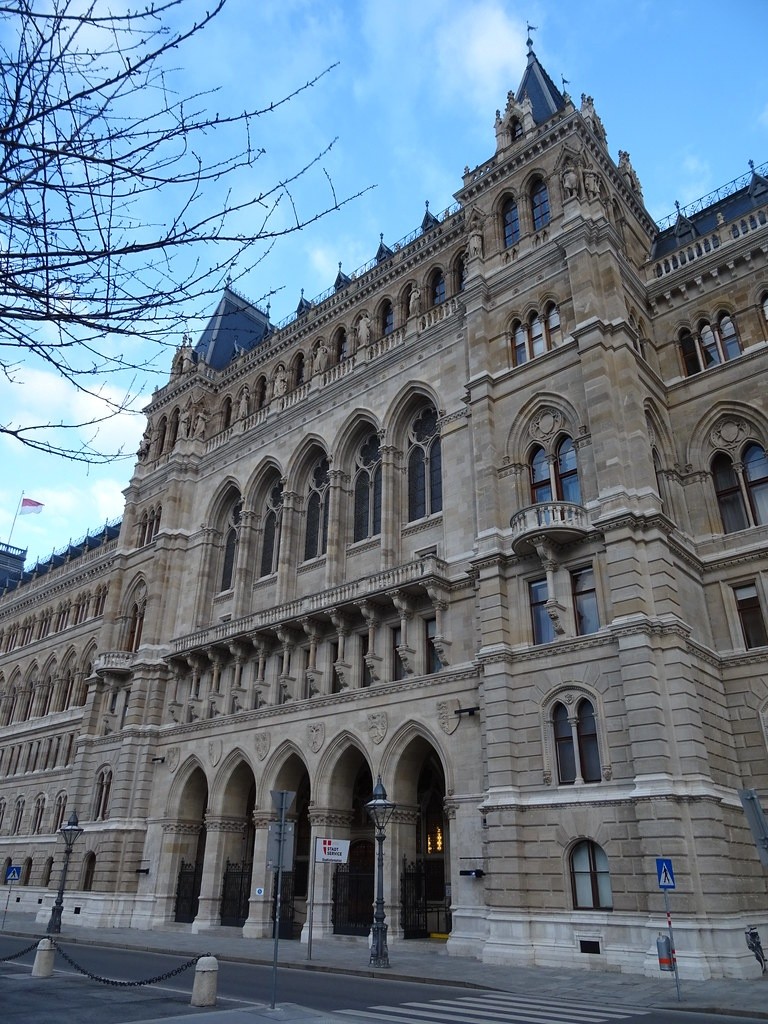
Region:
[363,774,399,968]
[46,808,84,934]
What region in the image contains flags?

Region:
[19,498,45,516]
